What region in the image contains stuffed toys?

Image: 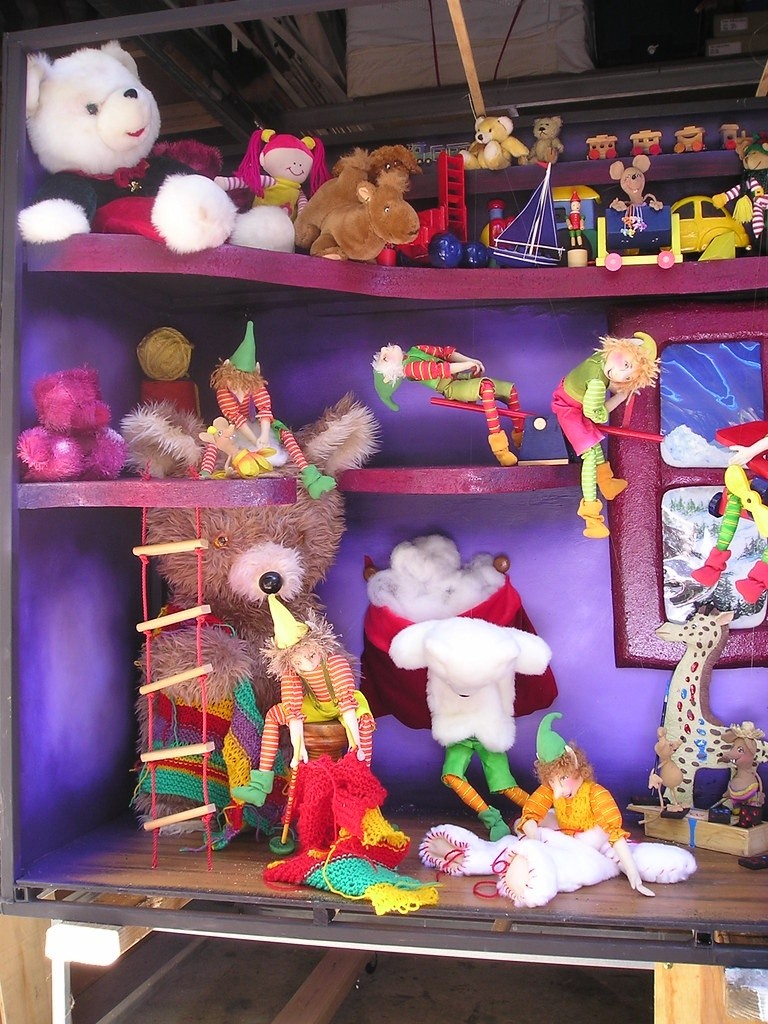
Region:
[418,808,697,907]
[215,130,332,221]
[18,39,295,251]
[17,366,128,480]
[519,117,564,164]
[458,117,529,170]
[713,141,768,256]
[123,391,381,830]
[722,723,765,815]
[656,605,768,807]
[638,728,691,824]
[608,155,663,211]
[388,617,552,842]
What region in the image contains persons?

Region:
[552,333,659,538]
[565,191,585,249]
[690,434,768,604]
[232,594,374,807]
[373,344,525,466]
[199,320,338,498]
[517,713,655,897]
[293,146,422,260]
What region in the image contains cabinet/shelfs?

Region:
[0,1,768,973]
